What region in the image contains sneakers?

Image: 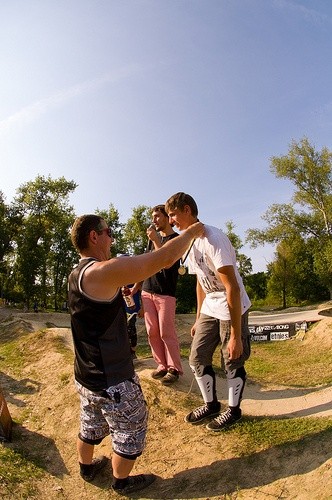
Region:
[151,367,179,384]
[206,407,242,431]
[79,455,109,483]
[111,473,156,496]
[184,401,221,423]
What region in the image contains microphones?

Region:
[147,225,154,250]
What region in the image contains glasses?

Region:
[94,227,114,239]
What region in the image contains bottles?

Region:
[121,286,136,310]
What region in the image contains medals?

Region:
[178,266,186,276]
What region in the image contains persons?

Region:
[121,204,184,387]
[66,214,205,496]
[165,192,253,431]
[117,253,142,361]
